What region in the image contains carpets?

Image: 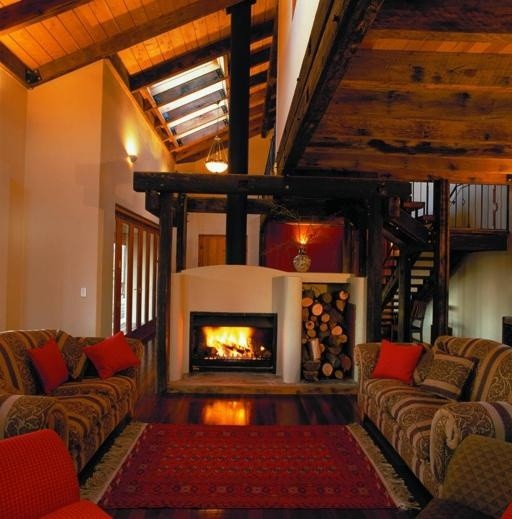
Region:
[82,420,419,515]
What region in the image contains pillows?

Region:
[28,334,69,389]
[413,340,449,388]
[369,336,424,385]
[421,349,478,402]
[53,330,92,384]
[87,329,140,378]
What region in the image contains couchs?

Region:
[1,327,146,484]
[354,336,511,495]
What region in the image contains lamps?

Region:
[204,99,229,175]
[122,136,138,164]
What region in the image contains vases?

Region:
[293,248,312,273]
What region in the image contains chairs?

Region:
[386,298,427,345]
[1,430,112,519]
[417,432,512,519]
